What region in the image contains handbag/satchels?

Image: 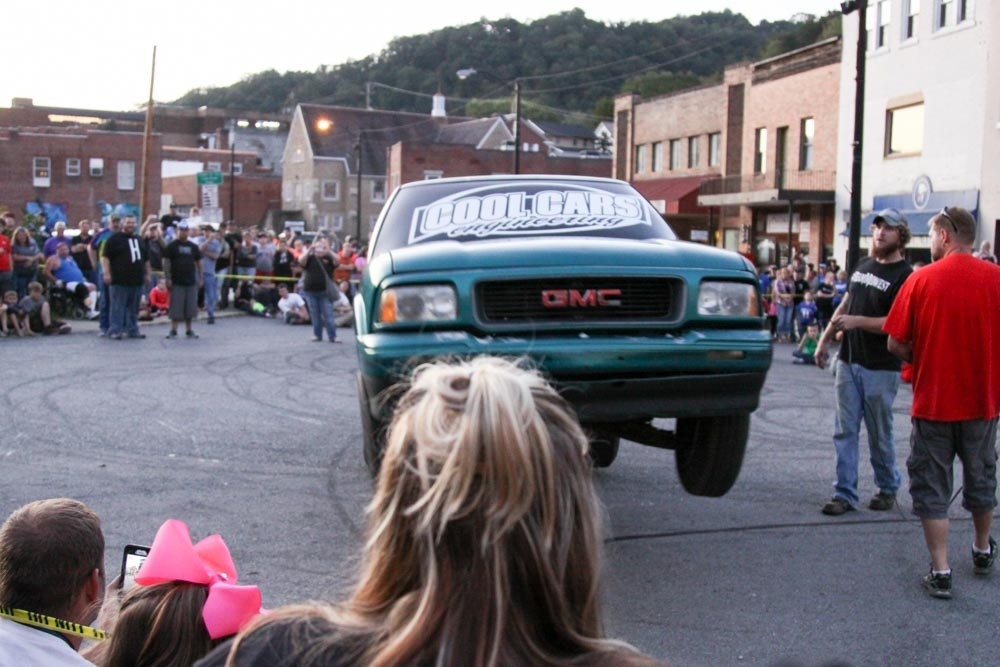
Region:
[326,280,341,303]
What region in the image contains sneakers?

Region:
[921,568,953,598]
[972,535,997,574]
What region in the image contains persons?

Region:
[0,202,366,336]
[732,240,851,366]
[882,206,1000,596]
[190,351,664,667]
[813,209,925,516]
[298,238,342,342]
[101,213,152,341]
[0,497,162,667]
[911,240,997,273]
[161,221,204,340]
[97,520,261,667]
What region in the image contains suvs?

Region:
[352,175,773,497]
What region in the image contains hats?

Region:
[872,207,909,228]
[177,219,189,229]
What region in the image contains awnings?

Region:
[839,209,979,238]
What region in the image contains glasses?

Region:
[940,206,958,235]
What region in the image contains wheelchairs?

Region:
[48,285,86,319]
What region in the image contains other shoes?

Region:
[330,337,341,343]
[127,330,146,339]
[110,334,121,340]
[0,309,100,338]
[166,332,177,339]
[821,496,849,514]
[869,492,896,509]
[186,331,198,339]
[207,314,214,324]
[312,337,322,342]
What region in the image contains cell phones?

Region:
[119,544,151,593]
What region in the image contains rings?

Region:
[836,325,841,330]
